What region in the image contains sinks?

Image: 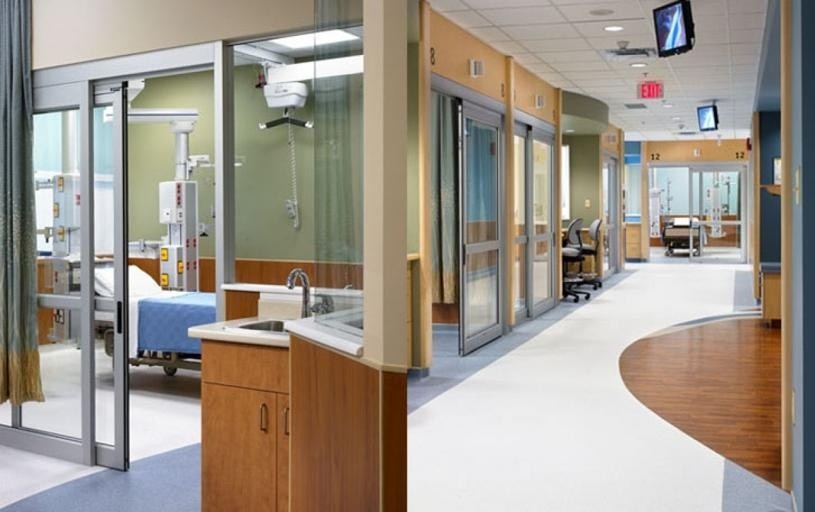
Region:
[238,320,290,333]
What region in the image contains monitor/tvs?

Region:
[696,106,719,131]
[652,0,695,58]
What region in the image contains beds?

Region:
[92,262,217,378]
[663,215,701,257]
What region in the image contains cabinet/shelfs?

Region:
[201,339,291,512]
[625,222,642,260]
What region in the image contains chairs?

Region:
[561,216,605,304]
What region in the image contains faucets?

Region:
[310,292,336,316]
[286,267,313,320]
